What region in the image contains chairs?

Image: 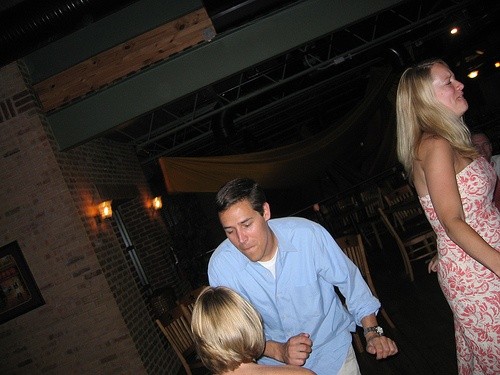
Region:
[378,208,437,282]
[335,234,396,353]
[384,185,434,259]
[331,187,394,249]
[156,285,211,375]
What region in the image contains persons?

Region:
[396,59,500,374]
[191,286,318,375]
[208,177,398,374]
[427,130,500,274]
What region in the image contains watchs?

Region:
[364,325,383,338]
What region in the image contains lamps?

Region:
[146,196,163,214]
[94,199,113,226]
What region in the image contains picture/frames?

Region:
[0,240,47,324]
